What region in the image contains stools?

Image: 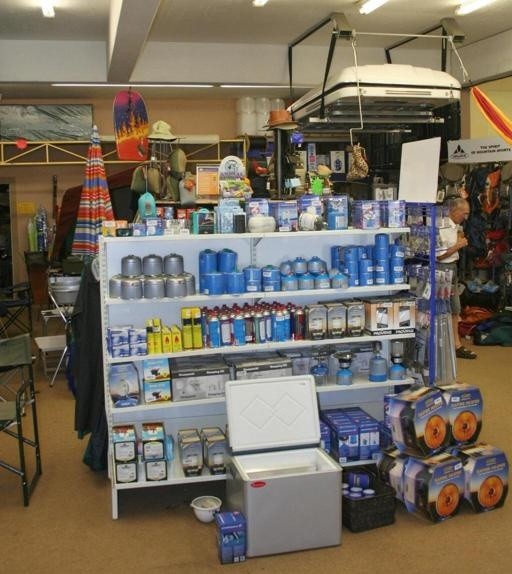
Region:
[33,335,69,374]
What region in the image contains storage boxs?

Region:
[377,381,510,523]
[224,374,344,557]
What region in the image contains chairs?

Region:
[0,285,42,506]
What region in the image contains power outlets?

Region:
[454,0,496,15]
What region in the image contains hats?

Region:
[261,109,299,131]
[144,120,177,142]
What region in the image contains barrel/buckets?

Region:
[235,97,256,113]
[256,98,271,112]
[271,98,286,111]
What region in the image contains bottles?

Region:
[200,302,305,348]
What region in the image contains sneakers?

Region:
[456,344,477,360]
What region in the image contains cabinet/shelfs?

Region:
[97,226,415,520]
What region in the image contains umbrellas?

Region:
[69,121,117,261]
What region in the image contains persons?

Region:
[436,197,479,360]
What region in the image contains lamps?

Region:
[358,0,389,15]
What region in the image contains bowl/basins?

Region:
[190,495,222,524]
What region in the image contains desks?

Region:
[24,250,50,301]
